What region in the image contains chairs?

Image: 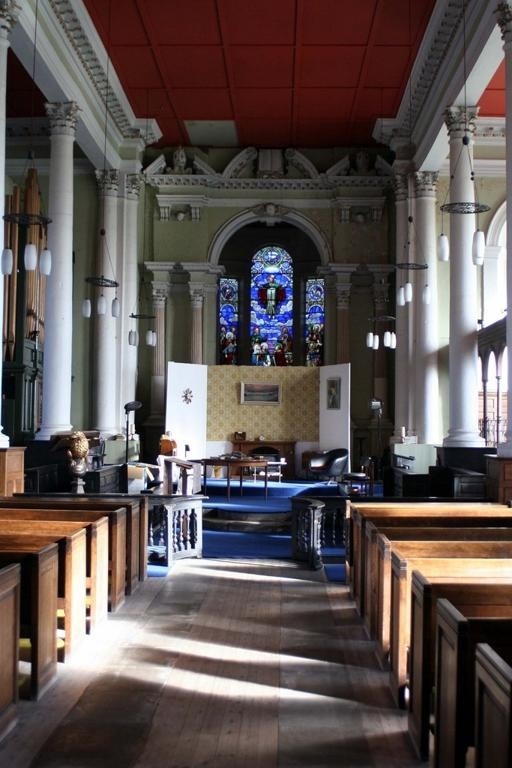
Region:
[248,446,287,484]
[304,448,348,481]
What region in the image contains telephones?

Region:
[232,451,246,458]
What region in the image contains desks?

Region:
[188,458,268,502]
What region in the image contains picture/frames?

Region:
[325,376,341,410]
[240,380,283,406]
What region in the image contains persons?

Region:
[220,321,324,366]
[262,273,280,319]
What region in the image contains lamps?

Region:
[435,0,491,267]
[129,0,158,348]
[82,1,121,319]
[396,0,431,306]
[0,0,54,275]
[365,0,396,350]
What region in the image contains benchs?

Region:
[1,490,148,738]
[345,496,511,767]
